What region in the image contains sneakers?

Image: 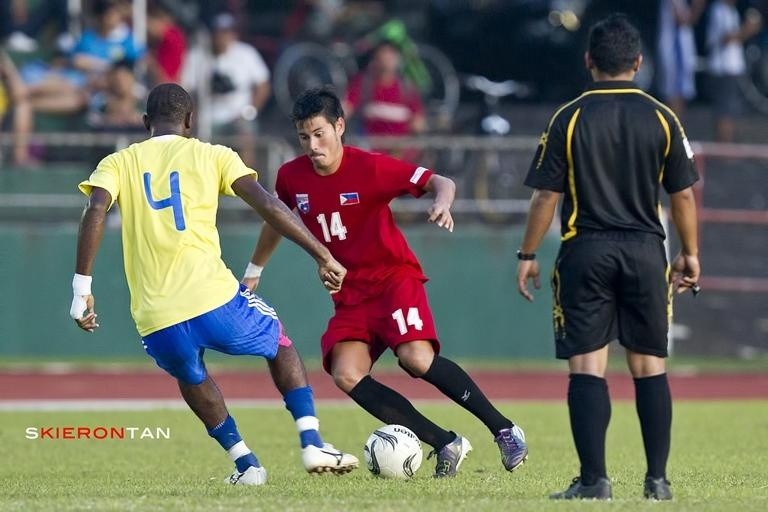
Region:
[491,419,531,473]
[642,475,674,503]
[547,473,614,501]
[432,430,475,478]
[219,465,271,485]
[298,440,362,477]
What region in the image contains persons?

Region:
[513,11,703,503]
[65,81,362,489]
[238,85,528,481]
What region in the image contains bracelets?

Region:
[241,262,264,279]
[515,247,537,263]
[70,274,94,297]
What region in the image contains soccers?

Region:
[364,424,423,482]
